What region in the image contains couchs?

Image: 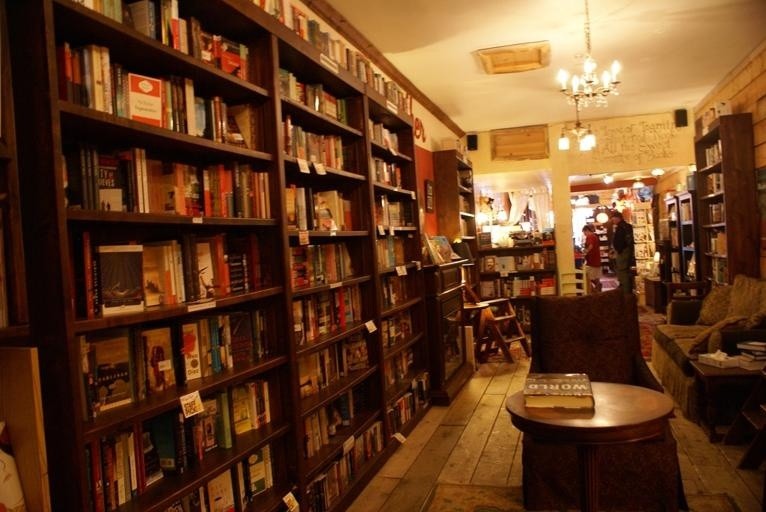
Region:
[651,273,766,420]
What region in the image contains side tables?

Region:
[687,352,765,443]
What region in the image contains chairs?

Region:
[527,286,690,512]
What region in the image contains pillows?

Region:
[535,287,643,384]
[689,274,765,354]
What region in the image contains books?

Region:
[81,0,250,84]
[522,371,595,410]
[308,421,385,511]
[382,310,413,347]
[670,118,731,292]
[76,233,259,320]
[168,444,272,512]
[422,232,458,264]
[477,225,558,313]
[78,312,270,419]
[278,69,345,169]
[374,196,404,228]
[294,285,361,345]
[304,388,354,456]
[389,373,432,434]
[385,346,414,384]
[252,0,407,113]
[289,244,352,290]
[737,337,766,369]
[86,379,269,511]
[57,45,262,149]
[369,119,401,188]
[63,148,270,219]
[382,274,408,310]
[377,237,403,269]
[297,332,369,396]
[286,184,352,230]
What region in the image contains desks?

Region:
[505,381,676,511]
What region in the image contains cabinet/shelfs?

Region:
[585,112,762,297]
[2,2,427,512]
[423,148,479,408]
[480,228,557,332]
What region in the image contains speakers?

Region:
[675,109,687,127]
[467,134,478,150]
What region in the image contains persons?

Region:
[607,213,634,294]
[581,225,602,292]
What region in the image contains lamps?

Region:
[557,0,622,107]
[558,94,597,151]
